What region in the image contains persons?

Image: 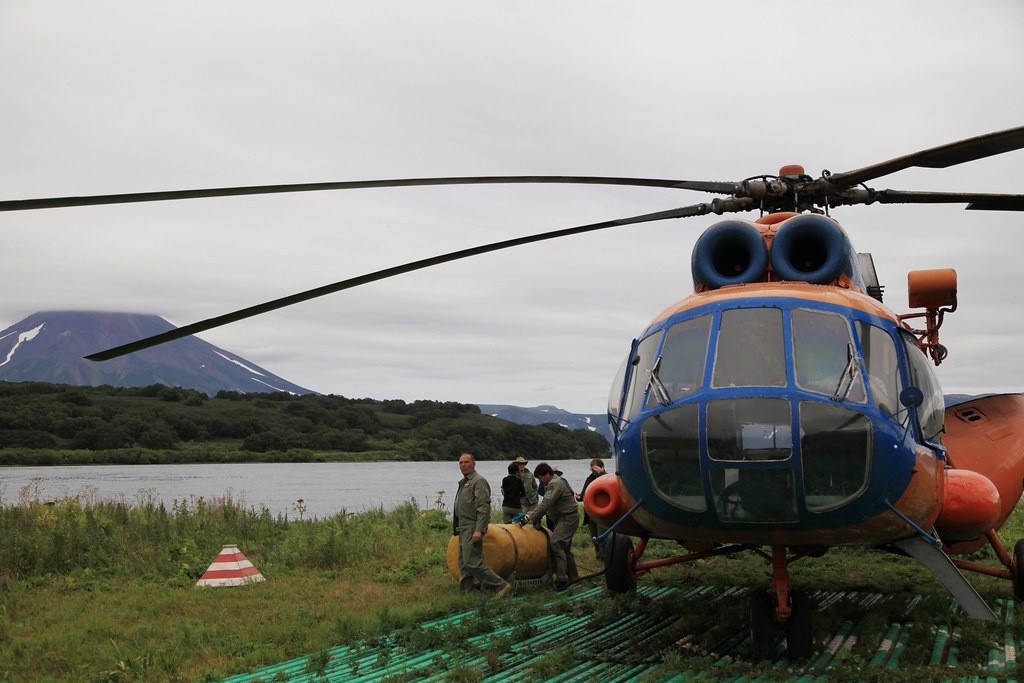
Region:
[575,458,610,560]
[501,456,580,589]
[453,453,511,600]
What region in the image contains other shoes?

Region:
[490,583,512,601]
[551,581,568,593]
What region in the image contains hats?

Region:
[551,464,564,477]
[513,457,529,464]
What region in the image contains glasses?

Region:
[519,463,525,465]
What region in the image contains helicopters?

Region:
[0,125,1024,622]
